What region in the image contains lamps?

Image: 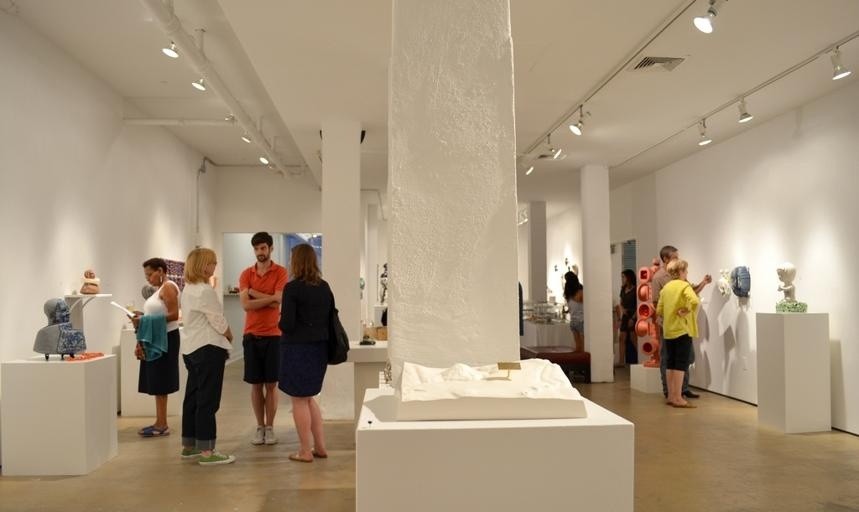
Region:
[162,23,278,171]
[516,0,724,176]
[610,31,859,170]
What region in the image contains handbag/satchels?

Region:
[324,305,351,365]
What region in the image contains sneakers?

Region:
[681,388,700,399]
[197,450,237,466]
[137,423,171,438]
[179,447,204,459]
[250,423,264,445]
[261,424,281,446]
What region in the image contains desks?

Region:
[347,340,387,445]
[523,317,576,348]
[120,328,188,417]
[355,387,635,512]
[0,354,118,477]
[756,311,831,434]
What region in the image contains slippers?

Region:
[288,448,315,463]
[665,398,698,409]
[311,445,330,458]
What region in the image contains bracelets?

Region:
[631,318,634,321]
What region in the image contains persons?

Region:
[615,269,638,368]
[777,262,795,301]
[652,246,711,399]
[240,232,288,445]
[656,259,699,408]
[278,243,335,462]
[180,248,235,467]
[564,272,583,351]
[129,258,181,437]
